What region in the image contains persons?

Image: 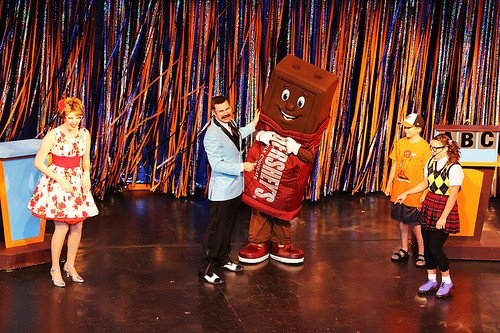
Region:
[380,113,432,267]
[199,93,257,285]
[27,97,108,288]
[394,132,465,299]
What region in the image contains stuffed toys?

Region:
[240,52,337,264]
